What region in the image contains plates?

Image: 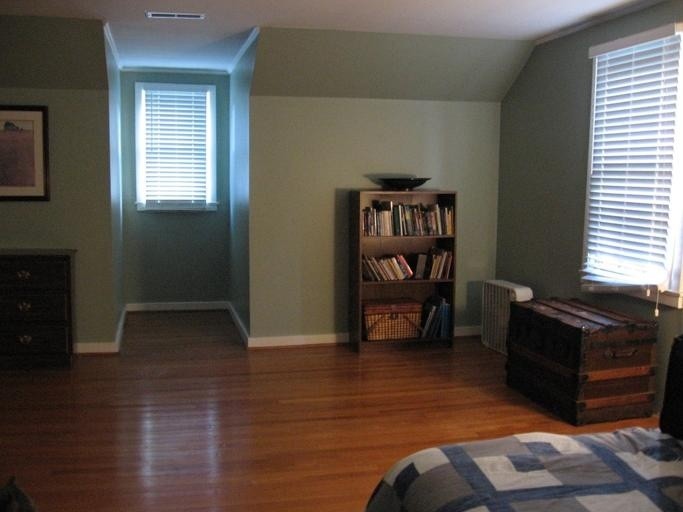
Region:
[378,177,430,191]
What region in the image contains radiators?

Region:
[480,279,534,357]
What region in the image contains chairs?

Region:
[0,475,35,512]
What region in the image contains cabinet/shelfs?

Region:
[348,188,458,352]
[0,248,78,368]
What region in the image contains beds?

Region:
[361,336,683,512]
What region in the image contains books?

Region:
[362,247,453,282]
[421,297,451,339]
[361,202,454,238]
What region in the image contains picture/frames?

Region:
[1,104,51,202]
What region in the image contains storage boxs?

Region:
[504,296,660,426]
